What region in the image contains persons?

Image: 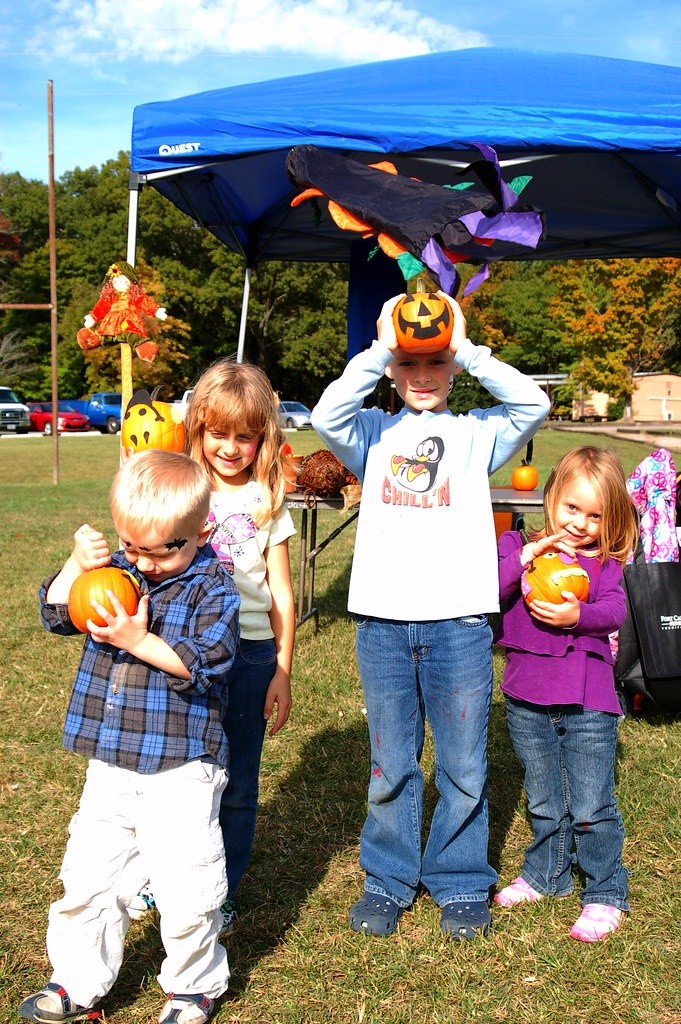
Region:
[494,445,641,946]
[122,358,299,935]
[16,448,244,1023]
[310,289,551,943]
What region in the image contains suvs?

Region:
[0,386,32,436]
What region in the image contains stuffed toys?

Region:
[75,259,169,365]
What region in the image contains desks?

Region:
[284,489,545,645]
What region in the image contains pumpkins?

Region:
[512,459,538,490]
[122,384,186,456]
[392,278,453,353]
[68,567,143,636]
[521,552,589,608]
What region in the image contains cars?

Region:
[25,402,92,436]
[277,401,313,429]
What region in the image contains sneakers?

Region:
[125,881,156,921]
[493,876,569,907]
[569,903,627,943]
[18,982,100,1024]
[216,899,251,939]
[158,992,214,1024]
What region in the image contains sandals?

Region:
[439,901,492,941]
[347,891,400,937]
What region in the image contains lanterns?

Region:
[389,290,454,352]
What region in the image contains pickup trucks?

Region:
[58,392,121,435]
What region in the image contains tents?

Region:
[131,43,680,263]
